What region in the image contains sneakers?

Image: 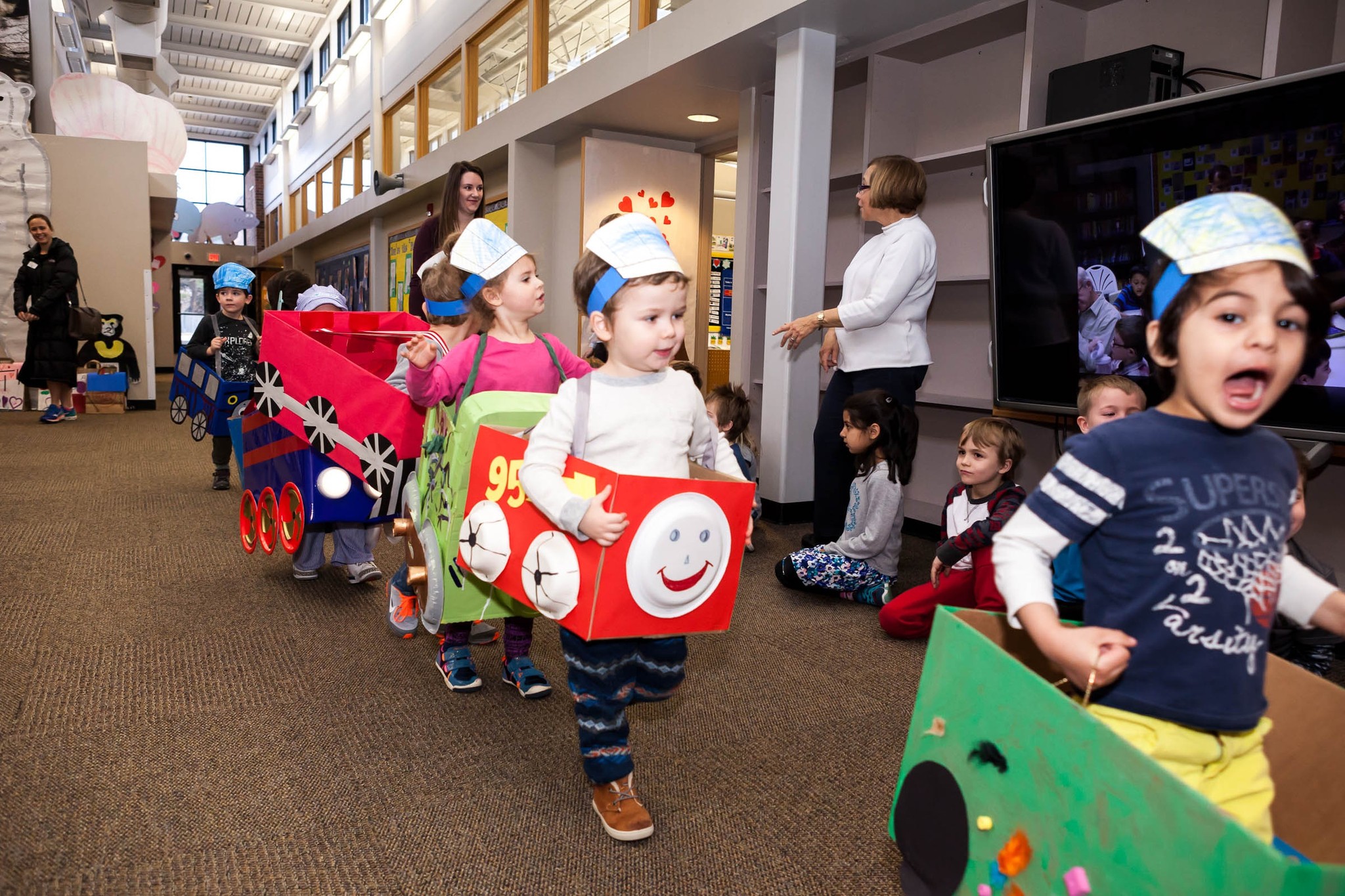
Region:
[858,584,892,605]
[212,468,230,489]
[347,559,382,585]
[63,408,77,421]
[592,771,655,842]
[292,564,318,580]
[40,404,65,423]
[435,620,500,644]
[385,576,418,639]
[502,654,553,698]
[434,639,483,693]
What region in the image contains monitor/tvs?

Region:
[985,62,1344,440]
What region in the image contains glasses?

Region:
[1112,337,1127,349]
[858,184,870,192]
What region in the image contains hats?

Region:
[293,283,349,312]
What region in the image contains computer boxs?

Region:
[1046,43,1184,127]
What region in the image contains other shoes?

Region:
[803,533,827,547]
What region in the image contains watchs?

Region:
[818,310,824,328]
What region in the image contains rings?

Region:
[790,336,795,341]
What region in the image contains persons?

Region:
[770,154,939,547]
[878,417,1028,639]
[185,276,263,491]
[1049,373,1146,616]
[990,186,1345,846]
[774,387,905,608]
[386,254,499,642]
[995,163,1344,389]
[408,159,486,323]
[668,356,768,558]
[398,231,603,702]
[13,213,91,423]
[518,214,757,843]
[293,285,384,583]
[1268,442,1338,660]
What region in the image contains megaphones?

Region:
[374,170,404,194]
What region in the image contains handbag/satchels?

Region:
[69,306,103,340]
